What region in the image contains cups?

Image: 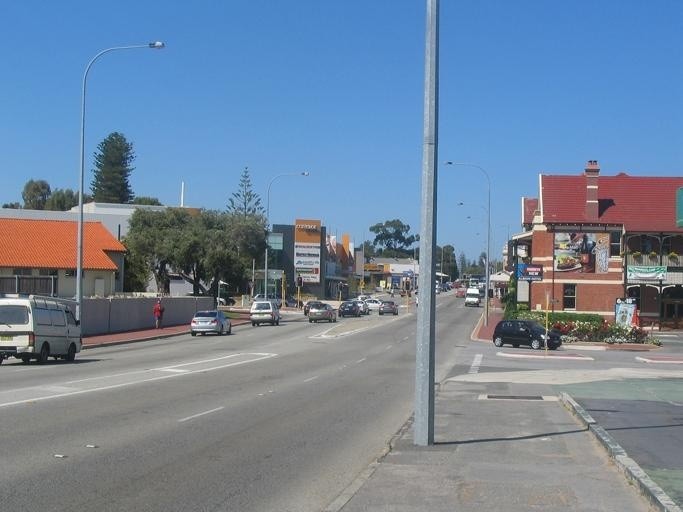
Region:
[593,239,608,273]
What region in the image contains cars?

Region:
[302,294,399,323]
[434,275,485,307]
[414,290,418,308]
[190,292,303,309]
[190,311,231,336]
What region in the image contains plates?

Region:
[553,256,581,271]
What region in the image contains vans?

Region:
[0,294,80,368]
[492,319,561,350]
[250,300,281,326]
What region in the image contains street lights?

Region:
[262,171,308,302]
[446,160,490,327]
[74,42,167,323]
[457,201,488,254]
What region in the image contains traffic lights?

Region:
[406,280,410,290]
[297,276,302,287]
[338,282,343,292]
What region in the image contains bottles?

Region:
[580,235,589,264]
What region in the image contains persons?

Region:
[520,324,525,331]
[153,300,165,328]
[390,287,394,298]
